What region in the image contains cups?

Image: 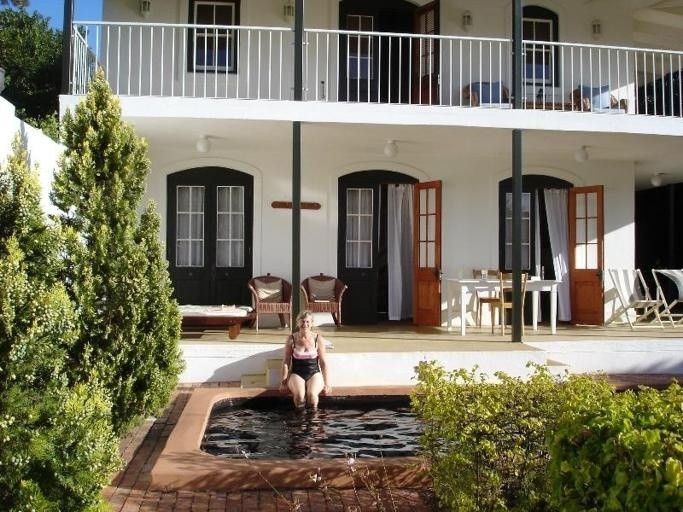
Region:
[480,269,489,280]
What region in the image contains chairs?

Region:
[246,273,347,333]
[473,269,529,334]
[606,268,682,331]
[463,82,629,113]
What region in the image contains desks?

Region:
[446,276,562,337]
[179,303,252,338]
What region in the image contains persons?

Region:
[278,311,331,409]
